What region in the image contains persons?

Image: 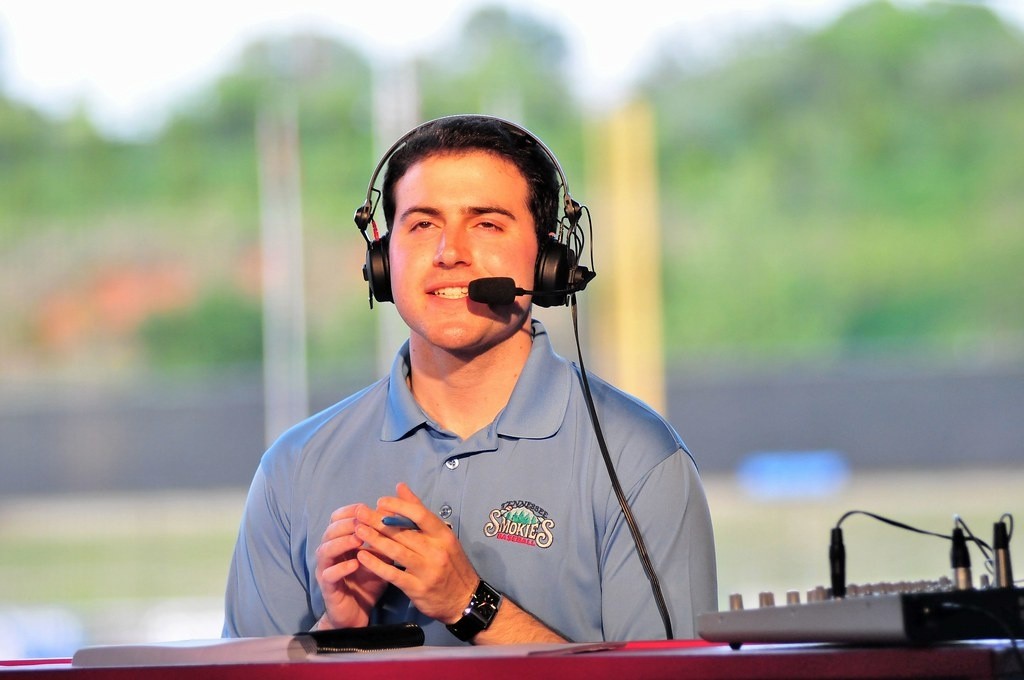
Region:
[220,118,718,646]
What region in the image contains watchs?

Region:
[444,577,502,644]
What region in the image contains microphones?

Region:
[468,276,586,304]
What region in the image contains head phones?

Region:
[354,113,597,310]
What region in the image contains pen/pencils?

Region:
[381,516,417,528]
